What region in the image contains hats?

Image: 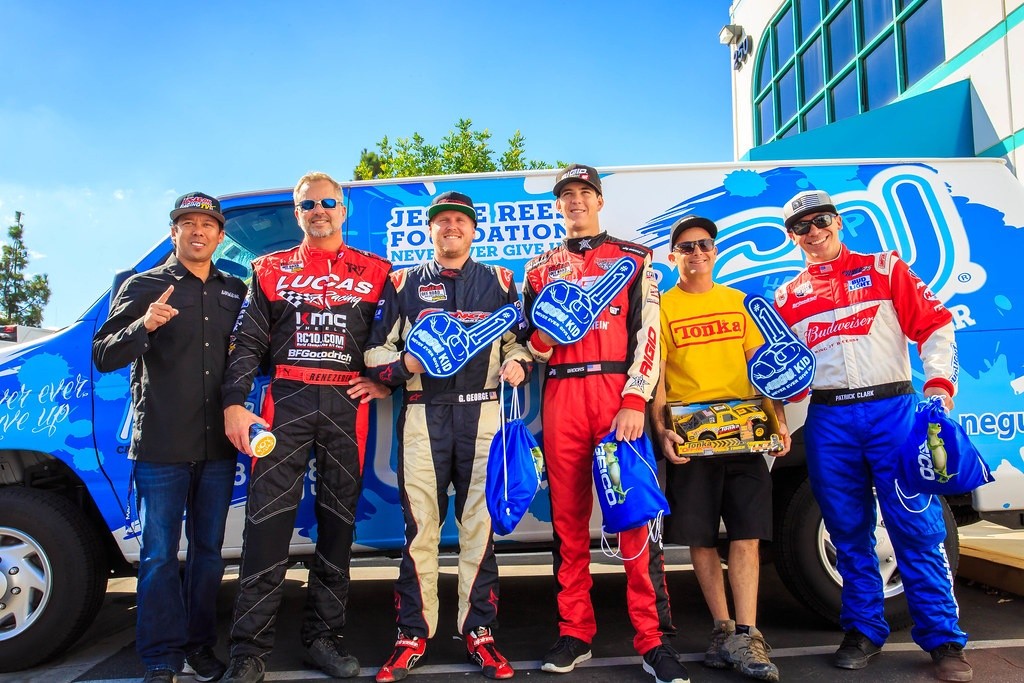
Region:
[669,215,718,253]
[552,164,603,197]
[782,191,837,228]
[170,192,227,225]
[427,191,477,221]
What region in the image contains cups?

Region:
[248,423,276,457]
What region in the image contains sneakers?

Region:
[182,649,227,681]
[140,669,178,683]
[642,644,691,683]
[302,636,361,678]
[224,655,265,683]
[705,619,735,668]
[541,636,592,673]
[720,626,780,682]
[375,632,430,683]
[457,627,515,679]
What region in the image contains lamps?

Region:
[718,24,742,46]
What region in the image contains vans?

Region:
[1,155,1024,676]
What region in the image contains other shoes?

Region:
[928,644,974,681]
[832,629,882,671]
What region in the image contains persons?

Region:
[363,189,534,683]
[221,172,401,683]
[92,192,251,683]
[743,189,973,682]
[516,163,692,683]
[645,216,791,683]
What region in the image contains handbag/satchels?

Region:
[593,429,671,562]
[896,394,996,513]
[485,375,542,537]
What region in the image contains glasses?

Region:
[787,212,836,235]
[295,198,343,212]
[673,238,715,255]
